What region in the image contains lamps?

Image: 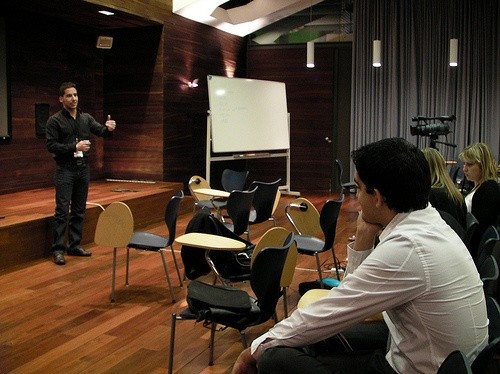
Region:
[449,0,458,66]
[307,0,315,68]
[372,0,382,67]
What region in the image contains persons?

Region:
[232,136,489,374]
[45,81,116,265]
[458,142,500,260]
[420,147,467,242]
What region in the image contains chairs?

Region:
[94,160,500,374]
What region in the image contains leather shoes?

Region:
[67,247,92,256]
[54,254,64,265]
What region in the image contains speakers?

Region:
[35,103,50,134]
